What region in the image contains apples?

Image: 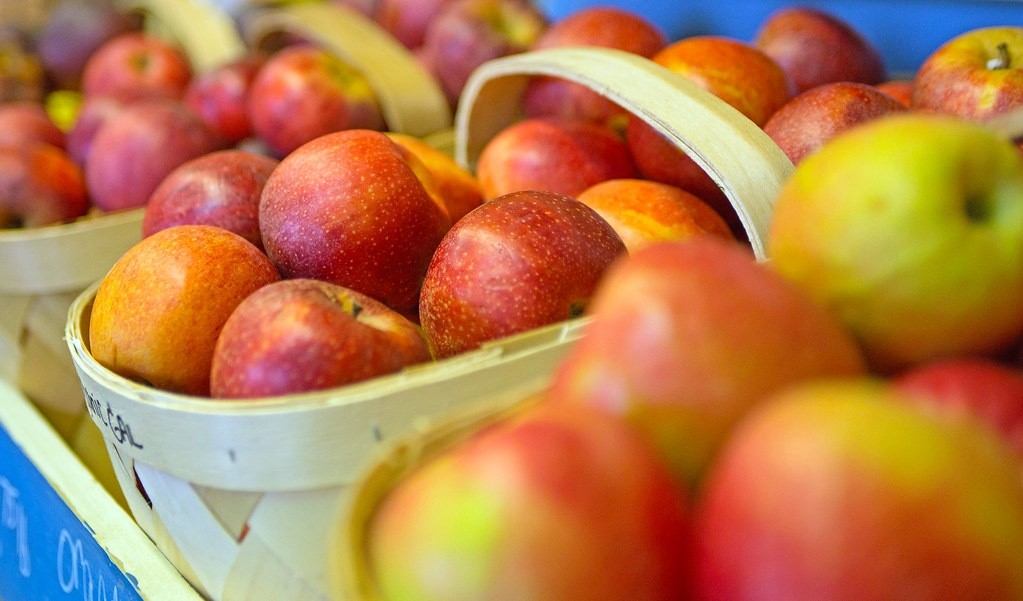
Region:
[0,0,1023,601]
